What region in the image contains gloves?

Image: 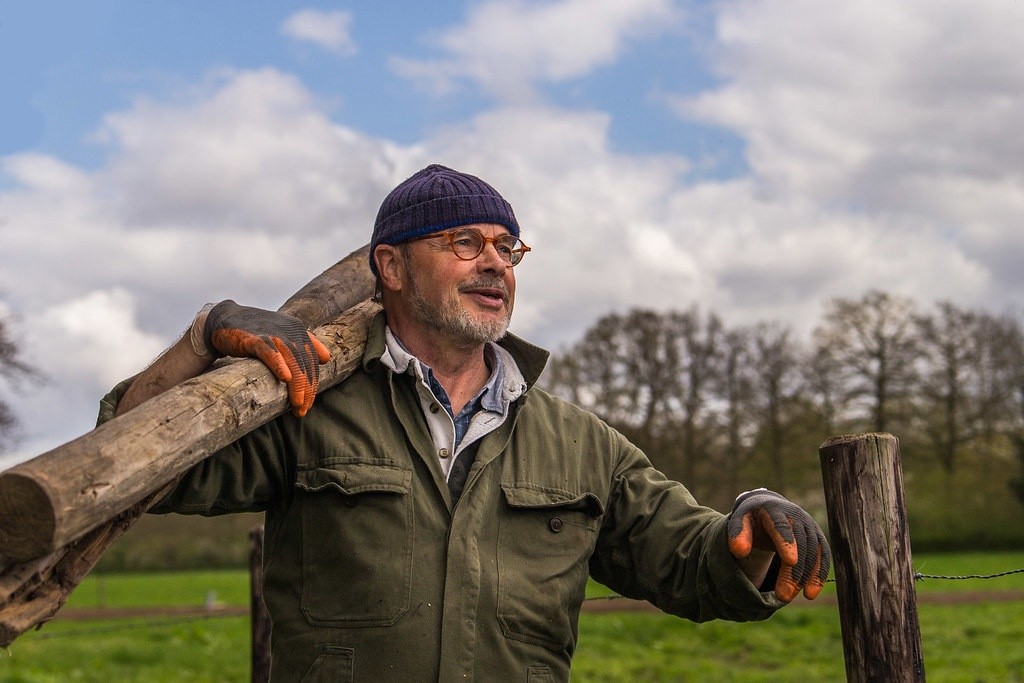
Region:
[204,299,331,419]
[728,489,832,604]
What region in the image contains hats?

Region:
[369,164,520,280]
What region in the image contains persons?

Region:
[94,164,831,683]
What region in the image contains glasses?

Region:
[394,228,531,268]
